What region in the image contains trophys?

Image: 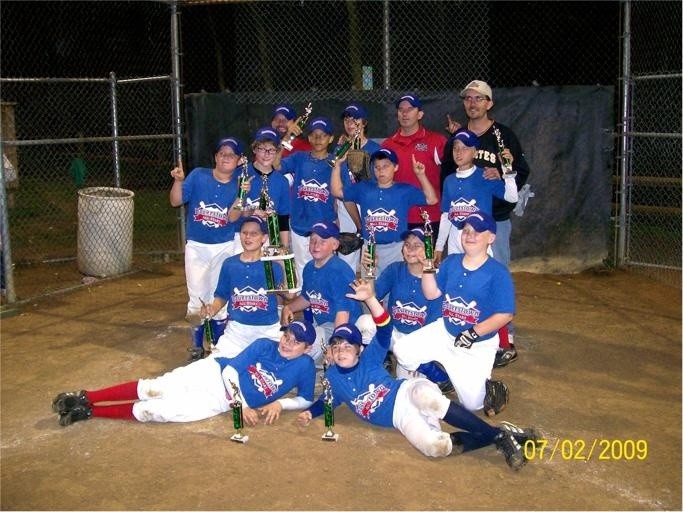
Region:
[228,381,249,444]
[320,377,338,442]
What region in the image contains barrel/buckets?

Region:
[76,186,136,278]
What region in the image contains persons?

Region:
[391,212,515,418]
[50,320,317,426]
[299,278,541,473]
[166,74,532,368]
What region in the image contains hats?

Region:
[280,320,316,346]
[243,215,268,234]
[216,136,241,154]
[450,128,481,149]
[400,226,424,243]
[304,223,339,241]
[255,105,396,162]
[329,324,362,344]
[396,91,422,110]
[457,212,497,232]
[460,79,492,102]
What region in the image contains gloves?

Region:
[453,327,478,350]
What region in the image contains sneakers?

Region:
[497,421,546,470]
[433,379,454,396]
[186,347,204,363]
[484,380,508,415]
[494,345,519,368]
[52,390,93,426]
